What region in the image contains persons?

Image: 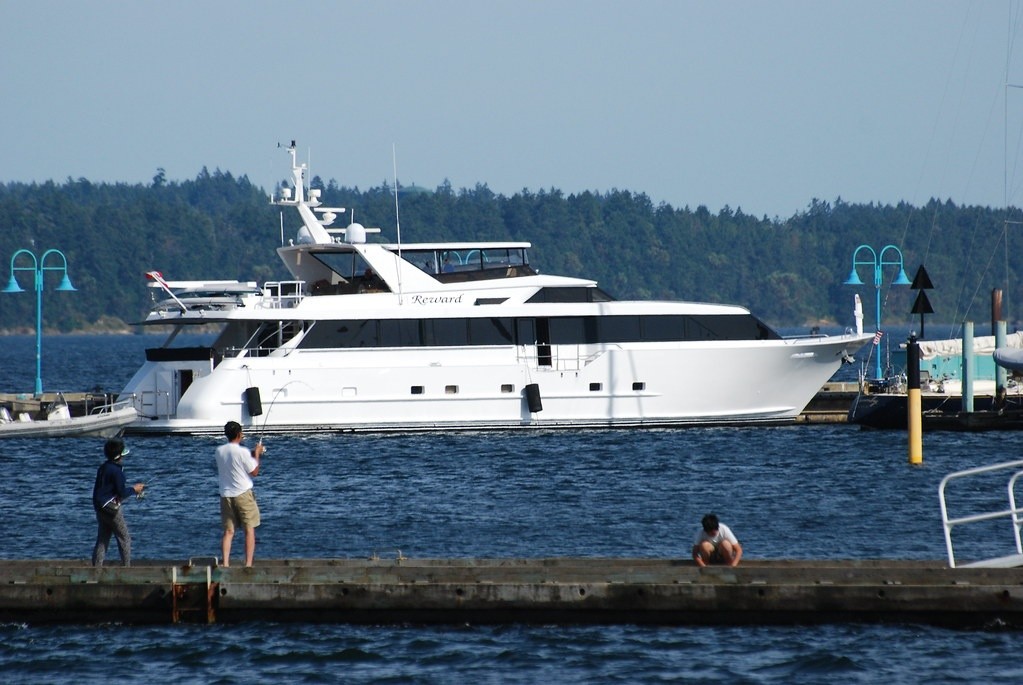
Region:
[693,514,743,568]
[215,419,262,568]
[987,387,1016,416]
[92,436,145,569]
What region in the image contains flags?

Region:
[874,331,882,345]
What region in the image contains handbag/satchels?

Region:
[102,498,122,517]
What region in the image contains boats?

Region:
[109,138,878,436]
[0,391,138,440]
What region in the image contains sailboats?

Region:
[846,0,1023,428]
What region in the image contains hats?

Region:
[103,438,130,460]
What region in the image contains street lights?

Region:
[0,248,79,400]
[843,245,914,380]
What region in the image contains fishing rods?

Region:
[130,433,223,505]
[253,379,313,458]
